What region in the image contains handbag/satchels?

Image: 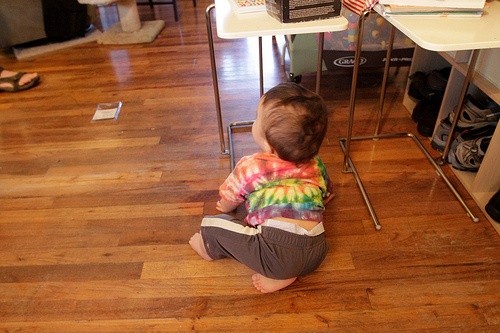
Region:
[265,0,341,24]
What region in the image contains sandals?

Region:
[0,69,40,92]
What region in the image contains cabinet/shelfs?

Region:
[402,43,500,235]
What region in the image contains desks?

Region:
[205,0,500,230]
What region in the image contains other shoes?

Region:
[407,66,451,137]
[486,189,500,222]
[431,93,500,172]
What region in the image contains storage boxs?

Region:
[266,0,344,25]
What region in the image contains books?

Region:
[91,101,122,121]
[379,0,485,18]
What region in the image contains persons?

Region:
[0,66,39,92]
[188,80,333,294]
